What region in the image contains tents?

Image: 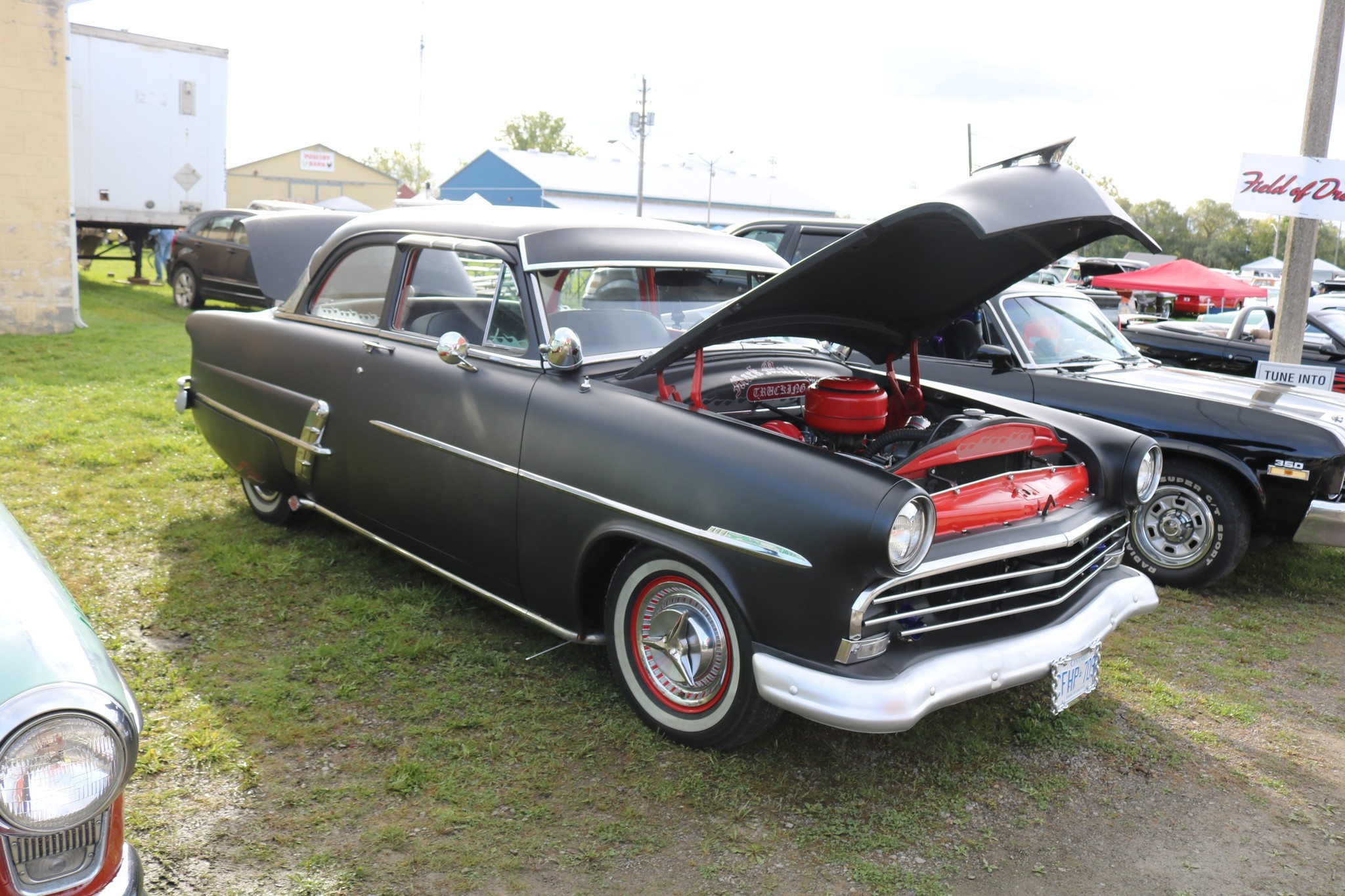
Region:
[1240,256,1284,276]
[1092,259,1268,325]
[1311,259,1345,283]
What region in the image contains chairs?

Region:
[545,309,669,356]
[409,308,524,345]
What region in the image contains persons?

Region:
[147,229,174,287]
[1309,283,1329,297]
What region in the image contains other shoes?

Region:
[154,278,162,282]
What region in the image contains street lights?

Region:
[607,140,644,216]
[1271,223,1279,258]
[689,150,734,228]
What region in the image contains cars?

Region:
[173,138,1165,755]
[581,220,1345,592]
[0,506,143,896]
[166,209,285,312]
